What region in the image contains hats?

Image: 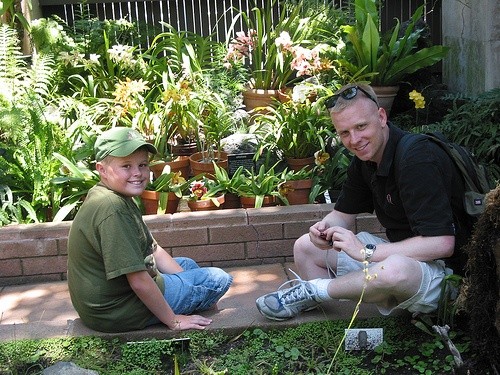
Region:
[93,127,158,161]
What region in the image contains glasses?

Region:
[325,86,379,111]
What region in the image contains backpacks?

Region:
[394,133,490,245]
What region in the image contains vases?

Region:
[188,193,226,212]
[187,193,225,211]
[277,88,317,103]
[239,82,283,114]
[151,155,189,181]
[189,150,228,178]
[140,190,182,215]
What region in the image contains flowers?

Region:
[75,27,334,166]
[139,166,188,216]
[189,172,225,207]
[405,86,429,110]
[310,150,355,205]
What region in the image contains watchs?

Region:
[364,244,376,265]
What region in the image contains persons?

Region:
[256,82,496,321]
[68,128,234,330]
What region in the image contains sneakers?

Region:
[255,270,330,322]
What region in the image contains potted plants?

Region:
[234,158,289,209]
[0,0,189,222]
[282,165,317,204]
[336,0,451,121]
